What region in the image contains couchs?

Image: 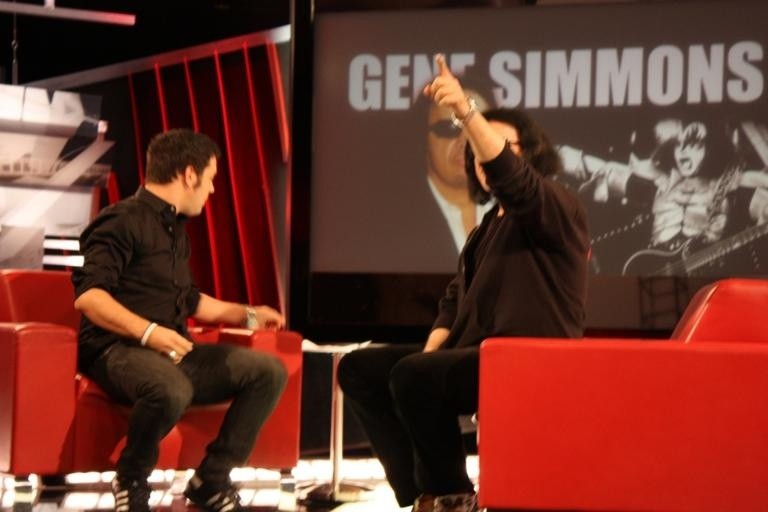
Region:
[0,268,304,511]
[476,278,768,512]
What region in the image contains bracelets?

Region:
[139,321,158,346]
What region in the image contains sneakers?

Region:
[182,473,246,512]
[433,492,484,512]
[110,477,153,512]
[409,494,432,512]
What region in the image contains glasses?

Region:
[428,119,461,138]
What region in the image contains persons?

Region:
[362,76,500,271]
[335,49,591,512]
[70,130,286,512]
[550,120,768,281]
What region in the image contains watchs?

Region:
[246,307,260,330]
[450,96,478,130]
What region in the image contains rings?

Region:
[168,350,177,358]
